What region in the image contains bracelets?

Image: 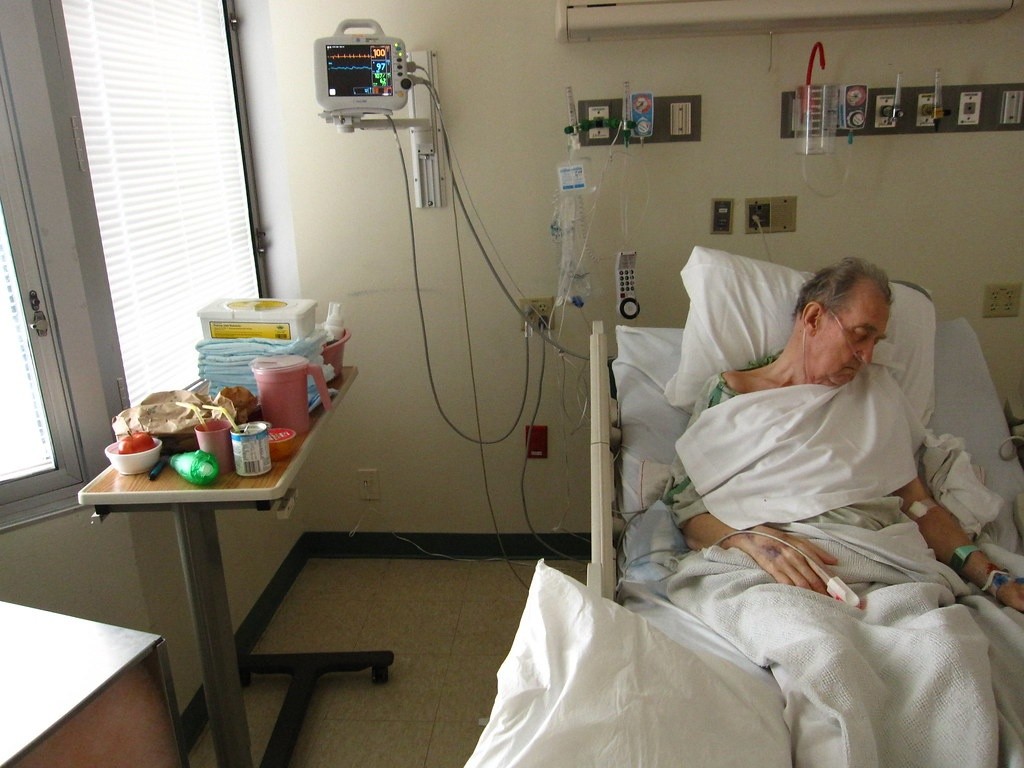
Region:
[950,544,984,572]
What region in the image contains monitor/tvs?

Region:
[325,44,394,96]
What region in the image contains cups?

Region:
[194,420,235,475]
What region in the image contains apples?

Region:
[118,430,157,454]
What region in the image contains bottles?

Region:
[168,450,219,487]
[325,301,345,346]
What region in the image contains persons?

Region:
[664,256,1024,626]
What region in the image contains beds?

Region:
[461,318,1024,768]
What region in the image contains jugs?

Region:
[249,355,333,436]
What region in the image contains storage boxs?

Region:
[199,295,320,348]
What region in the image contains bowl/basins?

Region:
[322,327,352,378]
[104,438,163,475]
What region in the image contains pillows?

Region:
[663,244,939,429]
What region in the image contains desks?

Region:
[76,363,394,767]
[0,599,189,768]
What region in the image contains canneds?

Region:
[230,422,272,476]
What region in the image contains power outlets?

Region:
[359,467,381,499]
[518,296,555,333]
[983,281,1020,317]
